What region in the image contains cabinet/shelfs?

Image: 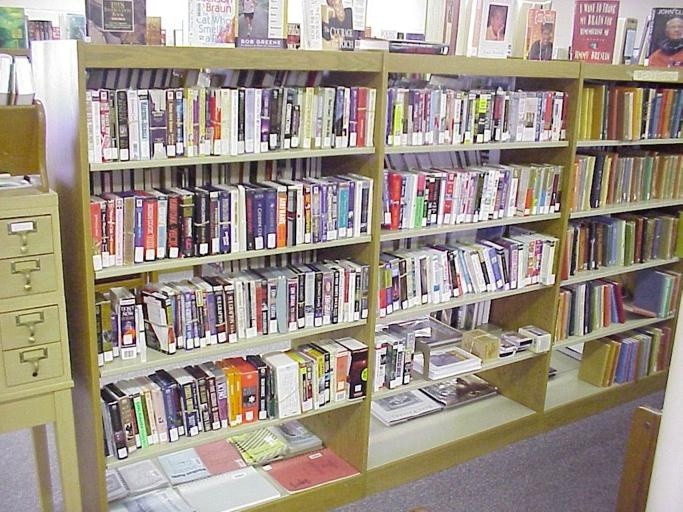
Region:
[2,184,81,510]
[542,60,683,425]
[26,38,383,512]
[361,52,580,511]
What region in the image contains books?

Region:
[370,88,569,428]
[83,83,370,512]
[86,0,683,70]
[550,84,683,386]
[0,3,82,56]
